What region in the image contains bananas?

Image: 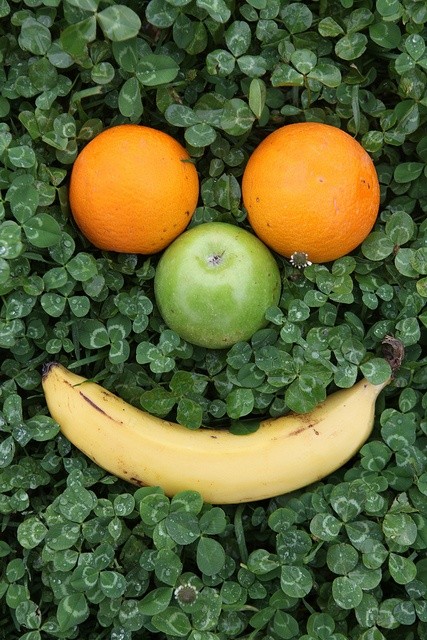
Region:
[38,336,404,507]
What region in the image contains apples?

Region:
[153,223,281,350]
